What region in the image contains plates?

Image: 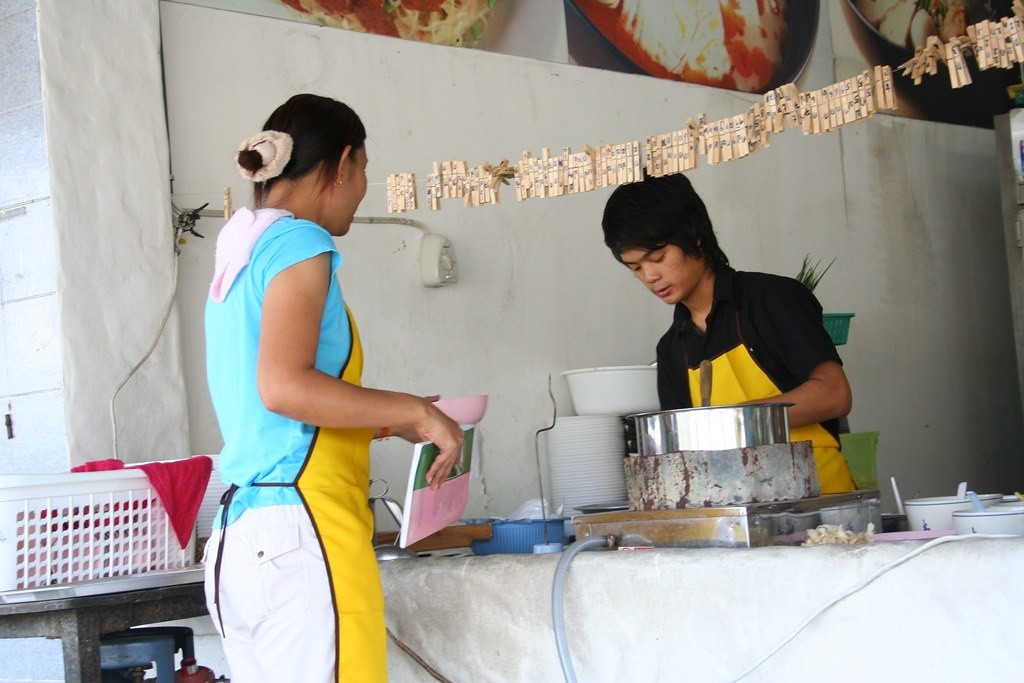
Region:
[545,415,628,519]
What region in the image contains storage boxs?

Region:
[0,454,214,591]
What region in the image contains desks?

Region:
[1,582,231,682]
[377,535,1021,682]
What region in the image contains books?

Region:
[399,424,475,549]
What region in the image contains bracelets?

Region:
[380,428,389,438]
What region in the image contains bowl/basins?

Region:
[431,394,487,428]
[1000,495,1024,505]
[573,501,629,514]
[559,365,661,416]
[951,506,1024,536]
[902,493,1003,531]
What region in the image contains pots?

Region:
[625,402,796,457]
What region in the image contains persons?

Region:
[197,95,464,683]
[599,164,859,496]
